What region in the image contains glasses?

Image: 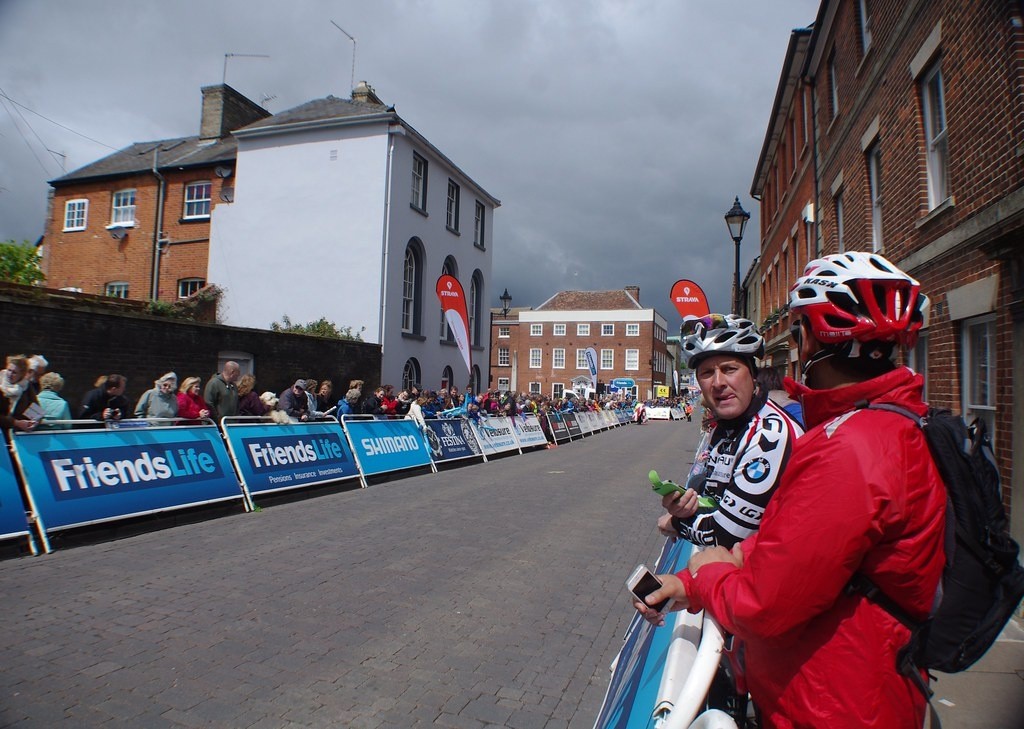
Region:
[161,383,173,388]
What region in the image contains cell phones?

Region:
[625,564,675,615]
[651,480,687,492]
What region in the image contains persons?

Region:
[174,376,209,426]
[238,354,804,462]
[79,374,134,429]
[134,371,178,426]
[632,252,948,729]
[203,361,240,424]
[0,353,48,433]
[658,313,806,548]
[36,372,72,429]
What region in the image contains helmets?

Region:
[790,249,932,361]
[678,312,762,368]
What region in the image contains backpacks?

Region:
[847,402,1024,676]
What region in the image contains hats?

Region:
[294,379,307,390]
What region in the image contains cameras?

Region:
[109,410,118,416]
